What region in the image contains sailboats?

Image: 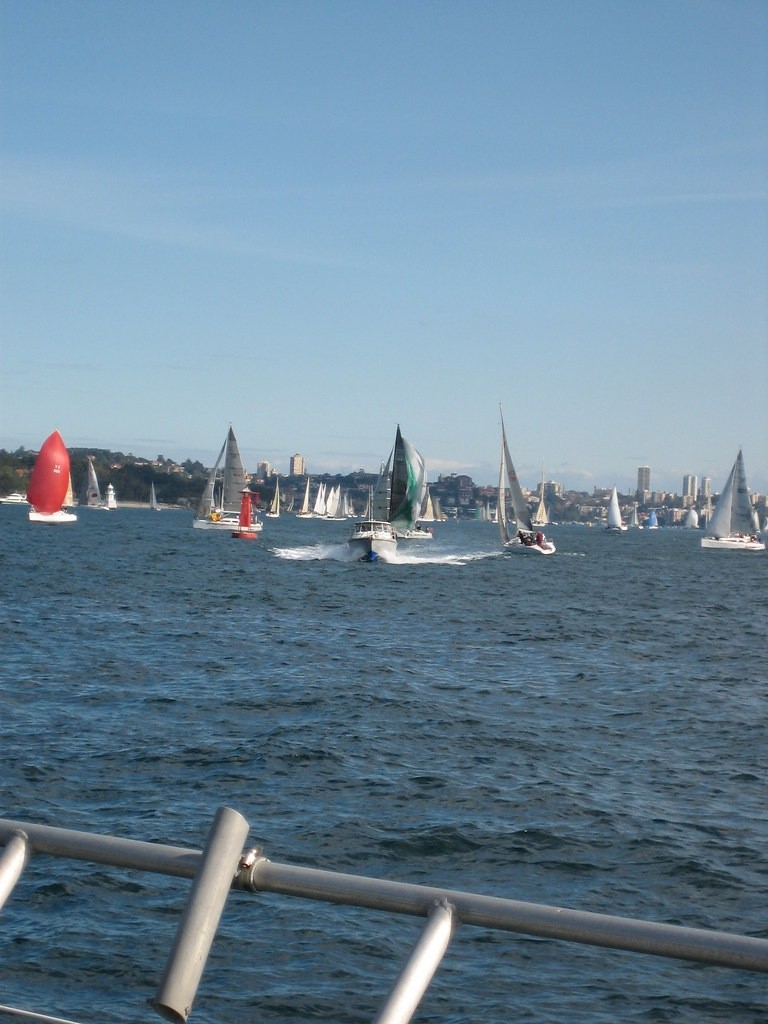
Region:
[193,420,264,533]
[80,455,110,511]
[150,481,161,511]
[683,510,700,528]
[372,424,435,540]
[347,484,398,565]
[701,443,768,551]
[265,476,369,520]
[626,501,643,530]
[646,509,658,530]
[496,402,556,555]
[26,430,77,522]
[601,482,623,535]
[231,487,257,540]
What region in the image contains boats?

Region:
[0,490,30,505]
